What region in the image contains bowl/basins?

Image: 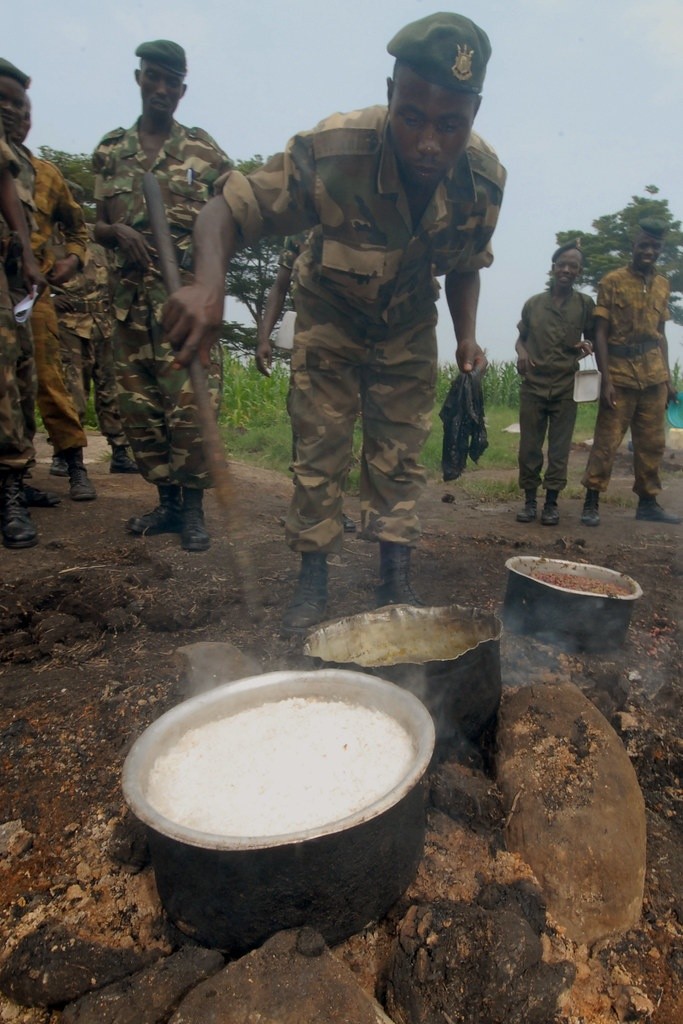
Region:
[667,391,683,428]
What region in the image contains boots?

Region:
[180,488,211,549]
[50,457,69,476]
[0,467,39,549]
[126,484,182,534]
[582,489,600,525]
[22,481,61,507]
[517,488,538,522]
[62,447,98,499]
[280,550,328,640]
[541,490,560,524]
[379,542,427,608]
[109,447,140,473]
[636,496,683,524]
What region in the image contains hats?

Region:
[638,217,671,240]
[0,57,31,89]
[387,12,491,94]
[63,178,85,206]
[551,237,585,263]
[136,39,187,76]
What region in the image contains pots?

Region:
[302,603,503,746]
[121,668,436,954]
[503,555,642,653]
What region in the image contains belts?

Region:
[608,341,658,358]
[71,301,110,313]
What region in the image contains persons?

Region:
[579,218,683,528]
[513,246,594,528]
[49,180,143,477]
[93,39,237,551]
[157,10,509,641]
[0,59,97,546]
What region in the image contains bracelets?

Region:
[581,340,593,353]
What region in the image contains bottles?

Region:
[274,310,298,350]
